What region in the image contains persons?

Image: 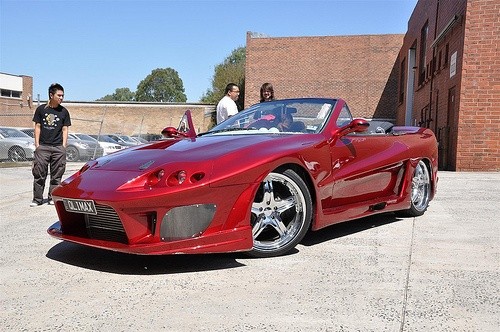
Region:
[217,83,241,130]
[253,82,275,120]
[30,83,71,206]
[274,113,293,133]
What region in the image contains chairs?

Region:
[294,122,305,132]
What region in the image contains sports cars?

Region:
[47,98,439,256]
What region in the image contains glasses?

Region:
[232,91,240,94]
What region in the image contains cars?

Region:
[0,126,36,162]
[68,134,163,156]
[17,127,104,162]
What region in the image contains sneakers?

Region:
[30,202,38,206]
[48,200,54,205]
